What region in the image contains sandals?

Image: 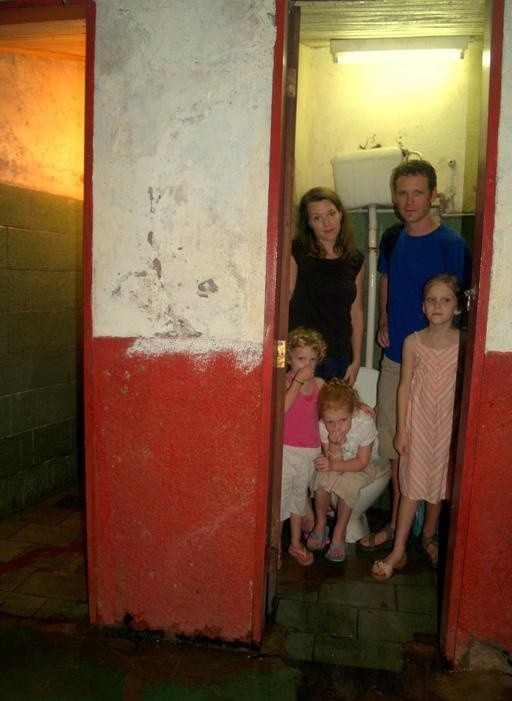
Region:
[424,538,442,565]
[357,525,394,551]
[372,556,408,580]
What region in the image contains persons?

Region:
[371,273,463,582]
[290,189,366,518]
[281,327,376,568]
[358,159,467,568]
[306,379,379,564]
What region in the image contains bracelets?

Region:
[293,378,304,386]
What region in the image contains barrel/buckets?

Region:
[329,145,406,544]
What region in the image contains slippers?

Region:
[288,509,346,566]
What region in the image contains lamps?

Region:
[330,37,469,65]
